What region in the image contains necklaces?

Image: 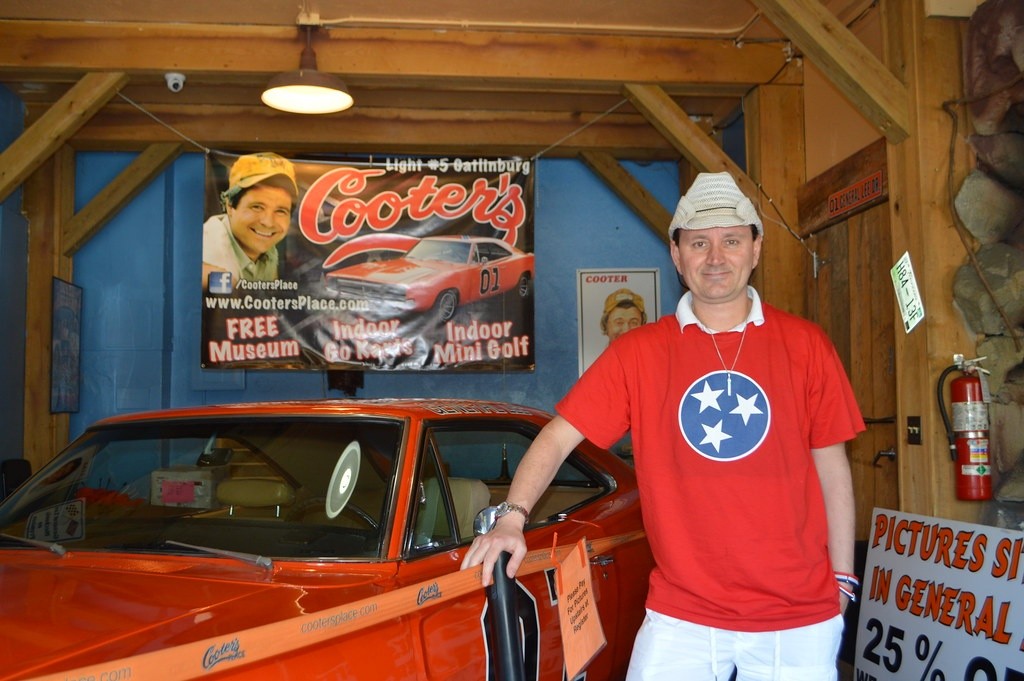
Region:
[691,299,750,396]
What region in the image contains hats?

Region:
[603,288,645,313]
[228,152,299,198]
[667,171,765,241]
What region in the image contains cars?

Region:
[1,393,654,681]
[326,232,538,324]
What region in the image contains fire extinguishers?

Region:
[937,353,993,501]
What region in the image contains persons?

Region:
[600,289,648,349]
[202,152,298,293]
[460,171,866,681]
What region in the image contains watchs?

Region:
[494,502,529,524]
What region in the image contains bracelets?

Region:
[833,571,860,603]
